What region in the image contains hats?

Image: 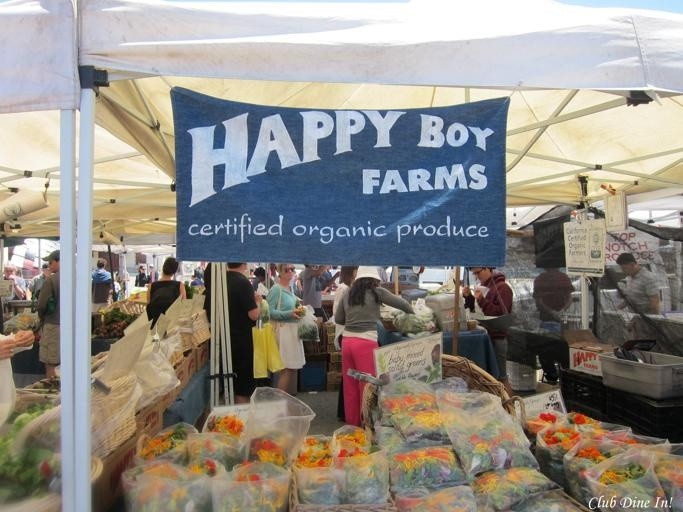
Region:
[354,265,381,282]
[40,249,60,264]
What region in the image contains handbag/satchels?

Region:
[262,319,286,374]
[250,311,269,380]
[29,277,55,315]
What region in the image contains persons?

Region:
[462,266,513,378]
[533,266,571,385]
[616,253,659,314]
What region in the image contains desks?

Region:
[393,325,499,377]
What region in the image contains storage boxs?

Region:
[566,340,619,378]
[321,321,345,393]
[423,293,467,333]
[601,348,683,401]
[295,354,329,392]
[88,338,209,512]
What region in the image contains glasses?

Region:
[280,268,296,273]
[471,268,484,275]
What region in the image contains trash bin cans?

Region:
[506,344,542,393]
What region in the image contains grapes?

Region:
[94,323,124,339]
